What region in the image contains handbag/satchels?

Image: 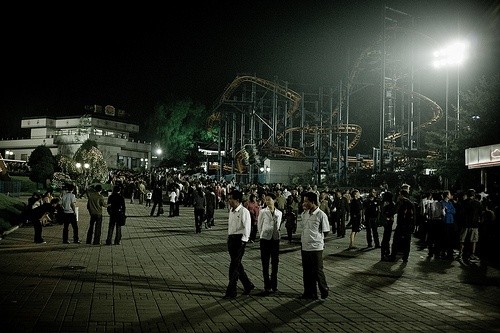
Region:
[160,207,164,214]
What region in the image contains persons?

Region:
[113,162,500,268]
[222,190,255,301]
[107,187,126,246]
[257,194,283,295]
[86,184,112,245]
[61,186,82,245]
[27,183,78,225]
[299,193,330,299]
[29,198,57,245]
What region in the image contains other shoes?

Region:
[33,240,46,246]
[368,245,381,250]
[168,213,179,217]
[63,240,82,245]
[336,235,345,239]
[157,214,161,217]
[301,294,318,300]
[86,241,101,245]
[241,284,255,297]
[221,293,238,300]
[106,241,120,245]
[194,227,201,233]
[150,212,154,216]
[402,259,408,263]
[349,244,356,249]
[263,287,278,295]
[321,288,330,299]
[382,254,393,261]
